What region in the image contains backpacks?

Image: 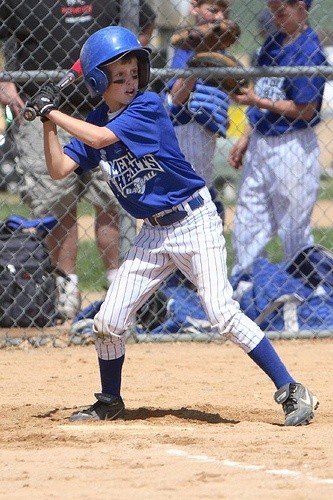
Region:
[0,222,71,329]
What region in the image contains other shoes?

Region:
[54,273,84,320]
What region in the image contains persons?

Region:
[22,25,319,426]
[0,0,328,319]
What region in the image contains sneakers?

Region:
[273,381,321,427]
[69,392,126,424]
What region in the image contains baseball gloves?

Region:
[169,19,241,52]
[186,52,255,96]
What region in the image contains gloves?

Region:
[25,81,62,123]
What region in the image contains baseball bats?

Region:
[23,58,84,122]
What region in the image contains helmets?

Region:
[79,25,153,99]
